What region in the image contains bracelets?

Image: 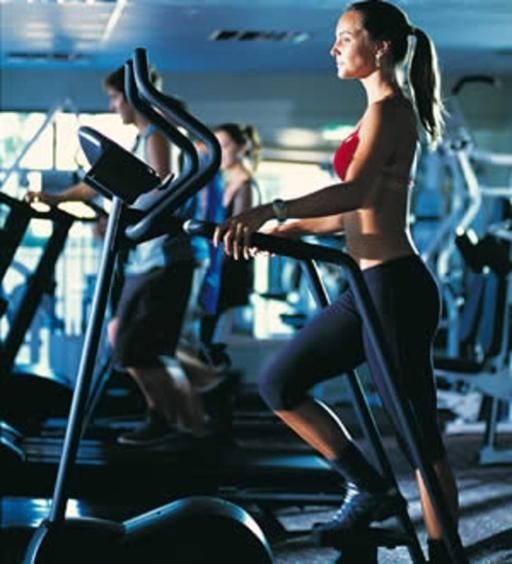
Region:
[273,196,290,224]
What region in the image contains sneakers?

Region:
[115,411,179,447]
[311,471,407,547]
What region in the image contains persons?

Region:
[20,61,217,449]
[210,0,469,561]
[195,120,267,369]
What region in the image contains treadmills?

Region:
[1,49,508,563]
